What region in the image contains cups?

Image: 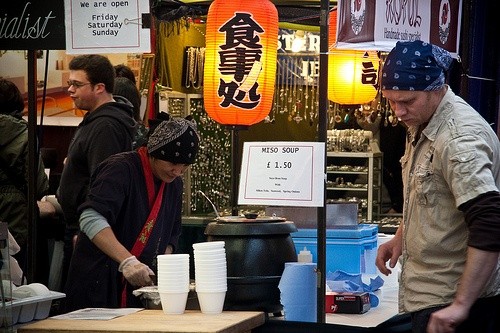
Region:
[157,254,190,313]
[193,241,228,313]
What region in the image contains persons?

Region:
[375,42,500,333]
[61,112,199,314]
[0,54,143,313]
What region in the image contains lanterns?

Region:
[327,10,379,105]
[204,0,278,124]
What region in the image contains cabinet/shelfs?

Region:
[327,151,383,223]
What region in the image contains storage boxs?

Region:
[288,220,380,286]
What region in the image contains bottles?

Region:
[297,246,312,263]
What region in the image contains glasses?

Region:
[67,80,92,88]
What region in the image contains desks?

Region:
[17,307,265,333]
[269,234,411,333]
